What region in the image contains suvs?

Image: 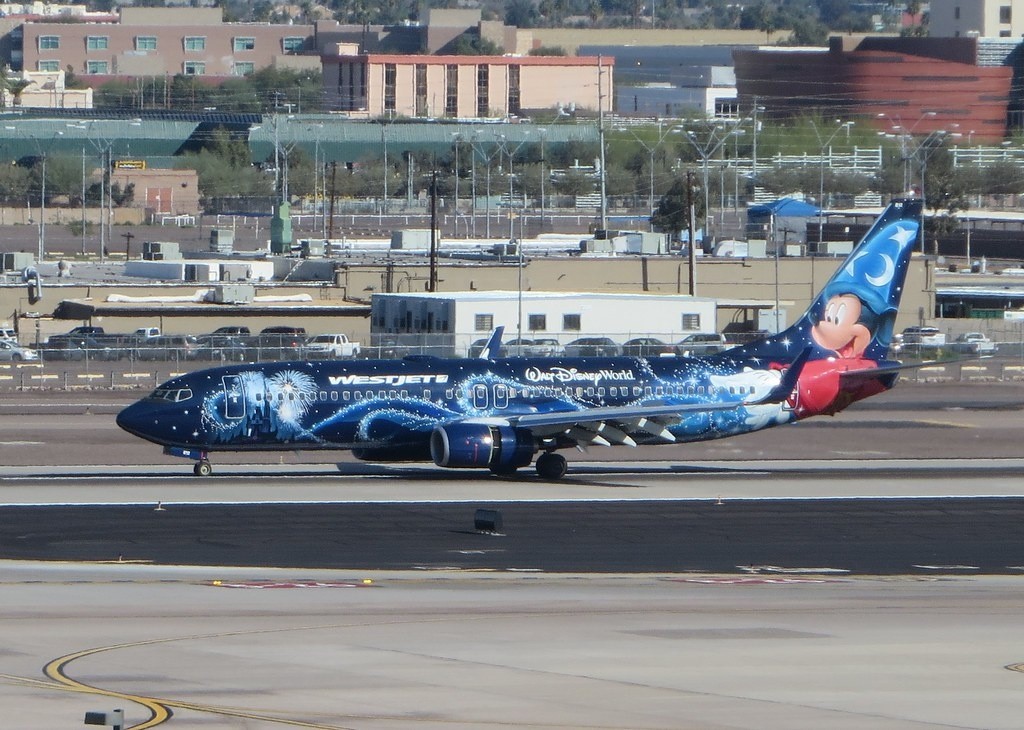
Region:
[677,333,727,355]
[0,329,18,349]
[901,327,946,344]
[247,327,307,360]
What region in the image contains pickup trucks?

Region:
[952,333,995,356]
[29,325,131,360]
[305,334,361,360]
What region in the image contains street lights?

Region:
[246,103,324,232]
[875,111,962,256]
[4,124,63,262]
[357,105,415,199]
[807,120,855,241]
[617,124,683,233]
[66,117,142,264]
[448,112,571,239]
[671,105,767,236]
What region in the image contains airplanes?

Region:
[115,195,927,482]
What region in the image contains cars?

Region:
[504,339,531,355]
[723,331,772,344]
[523,338,565,357]
[0,338,40,362]
[621,337,680,356]
[468,338,509,358]
[579,338,618,357]
[131,325,250,360]
